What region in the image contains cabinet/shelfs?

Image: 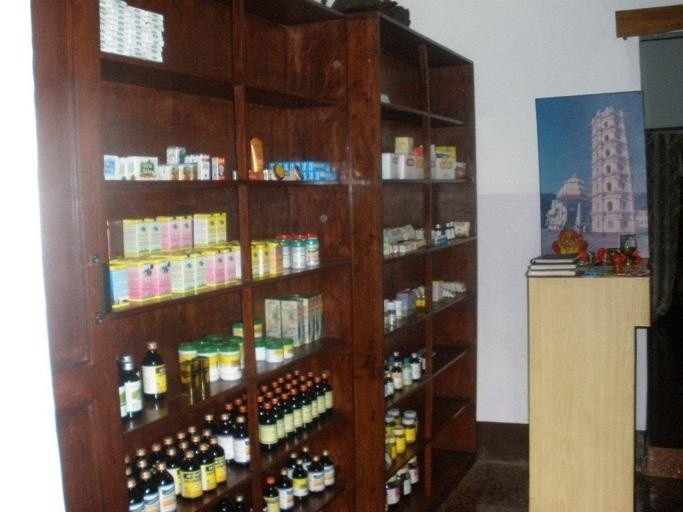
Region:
[355,10,476,511]
[529,263,651,512]
[30,0,356,512]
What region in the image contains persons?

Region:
[625,237,636,247]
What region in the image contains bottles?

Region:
[177,319,293,382]
[382,297,402,333]
[383,350,426,397]
[385,455,420,512]
[431,224,441,241]
[383,408,417,462]
[114,354,337,511]
[594,247,640,273]
[444,221,455,240]
[141,342,167,401]
[274,233,320,269]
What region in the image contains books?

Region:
[526,270,585,276]
[530,253,580,263]
[528,264,579,270]
